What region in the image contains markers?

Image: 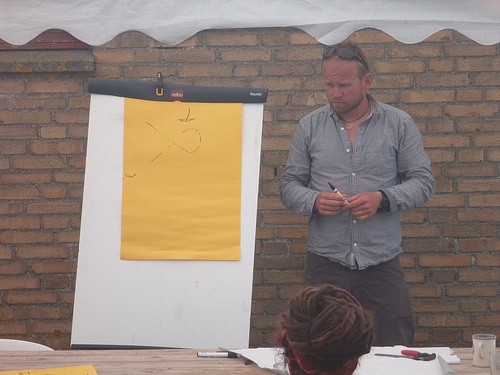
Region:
[196,352,241,358]
[328,181,349,206]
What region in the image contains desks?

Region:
[0,348,500,375]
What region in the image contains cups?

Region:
[489,347,500,375]
[471,333,496,367]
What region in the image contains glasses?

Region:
[322,47,364,66]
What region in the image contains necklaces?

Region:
[341,101,370,129]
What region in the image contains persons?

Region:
[274,282,374,375]
[281,42,436,347]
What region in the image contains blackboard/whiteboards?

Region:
[68,79,268,349]
